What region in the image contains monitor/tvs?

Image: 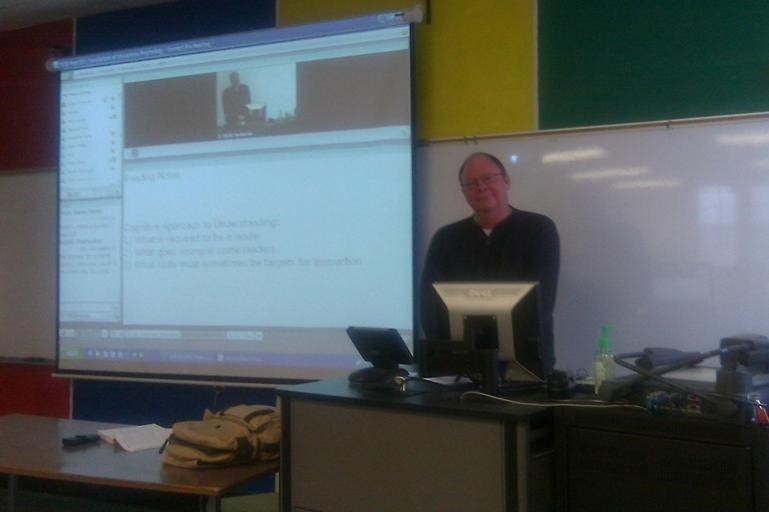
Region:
[417,279,550,394]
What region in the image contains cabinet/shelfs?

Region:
[553,383,769,511]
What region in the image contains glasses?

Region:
[461,172,503,193]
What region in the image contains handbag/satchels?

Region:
[161,404,282,470]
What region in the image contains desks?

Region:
[1,413,280,512]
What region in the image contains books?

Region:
[98,422,173,451]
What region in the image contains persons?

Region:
[223,71,251,124]
[419,151,561,376]
